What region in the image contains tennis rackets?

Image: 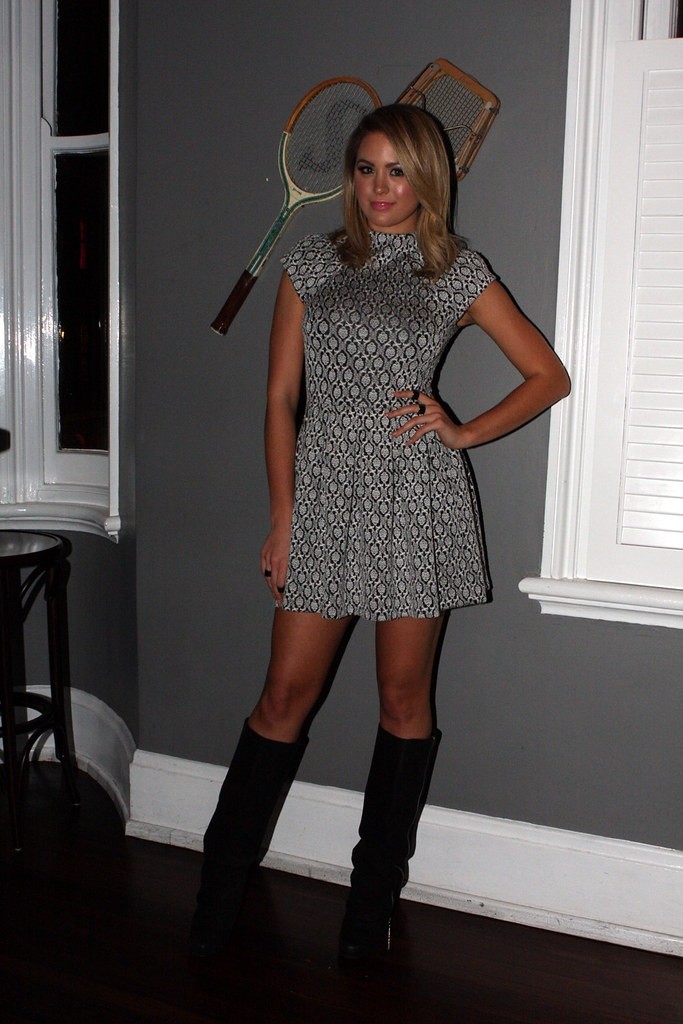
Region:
[211,75,384,336]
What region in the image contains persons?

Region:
[188,105,571,965]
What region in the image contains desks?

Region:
[0,529,82,851]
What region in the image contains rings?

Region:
[417,404,426,415]
[264,568,271,577]
[411,390,420,400]
[278,585,285,593]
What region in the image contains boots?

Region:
[340,722,443,977]
[190,717,309,956]
